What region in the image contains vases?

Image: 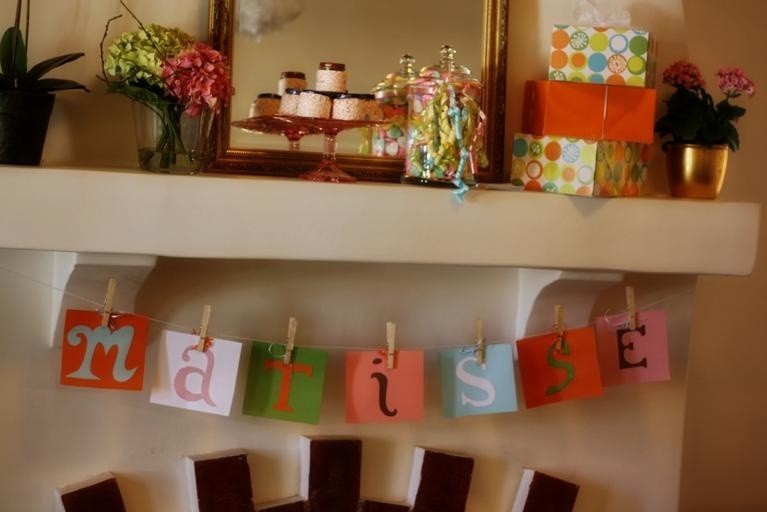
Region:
[663,143,728,200]
[131,99,214,175]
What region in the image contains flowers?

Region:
[653,59,755,153]
[95,1,234,172]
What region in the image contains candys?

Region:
[361,71,481,182]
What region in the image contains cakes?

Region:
[249,62,378,121]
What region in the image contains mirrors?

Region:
[202,2,509,186]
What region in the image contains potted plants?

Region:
[0,1,91,167]
